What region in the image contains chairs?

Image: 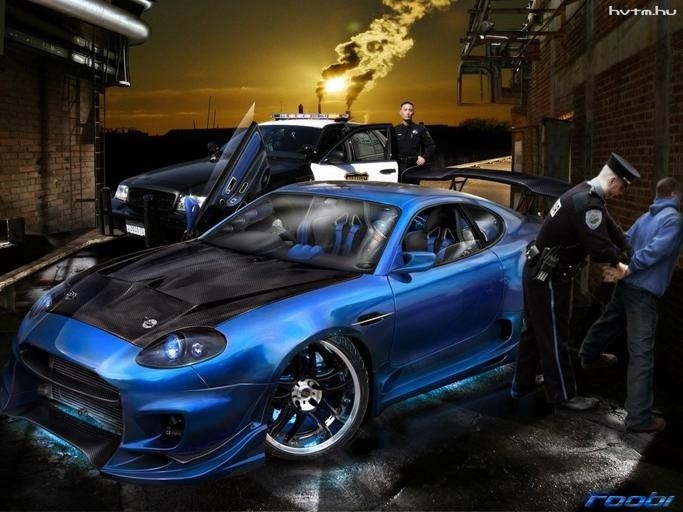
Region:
[294,198,477,280]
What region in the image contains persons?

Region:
[509,151,642,411]
[599,176,682,433]
[381,100,437,186]
